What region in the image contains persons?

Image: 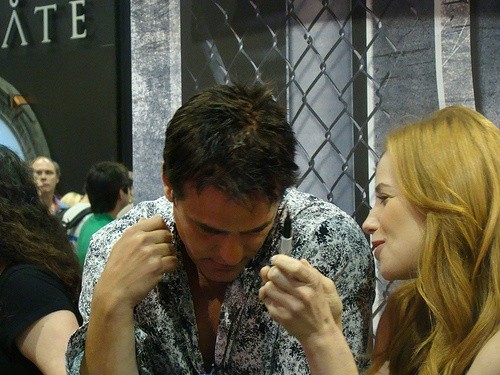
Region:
[0,143,83,375]
[30,155,133,269]
[64,71,377,375]
[258,104,500,375]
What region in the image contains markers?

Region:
[279,211,292,256]
